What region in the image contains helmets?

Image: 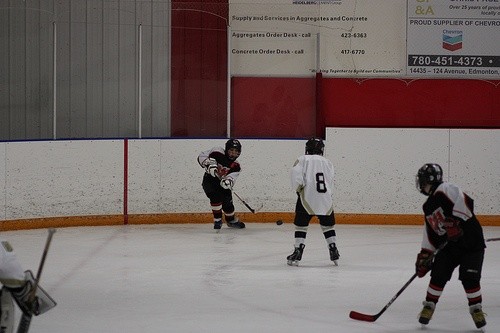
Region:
[305,137,325,155]
[415,164,444,196]
[225,139,242,161]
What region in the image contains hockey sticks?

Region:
[15,227,56,332]
[348,253,438,322]
[214,172,264,215]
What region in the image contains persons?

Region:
[0,240,38,333]
[197,140,245,233]
[416,163,486,333]
[287,138,340,266]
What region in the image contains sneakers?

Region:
[286,243,306,267]
[468,304,487,333]
[328,243,340,266]
[214,221,223,233]
[418,300,436,331]
[226,219,245,228]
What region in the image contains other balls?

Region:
[276,219,281,225]
